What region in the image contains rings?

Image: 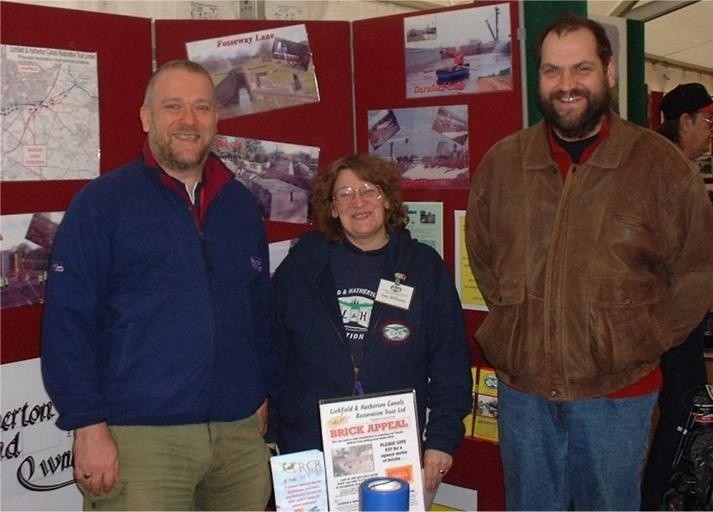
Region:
[439,469,444,473]
[82,473,90,479]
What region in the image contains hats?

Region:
[659,82,712,120]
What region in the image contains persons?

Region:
[459,13,712,510]
[642,79,712,441]
[257,151,475,511]
[35,57,277,511]
[454,46,464,67]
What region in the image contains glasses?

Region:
[334,184,385,204]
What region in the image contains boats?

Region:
[435,67,469,83]
